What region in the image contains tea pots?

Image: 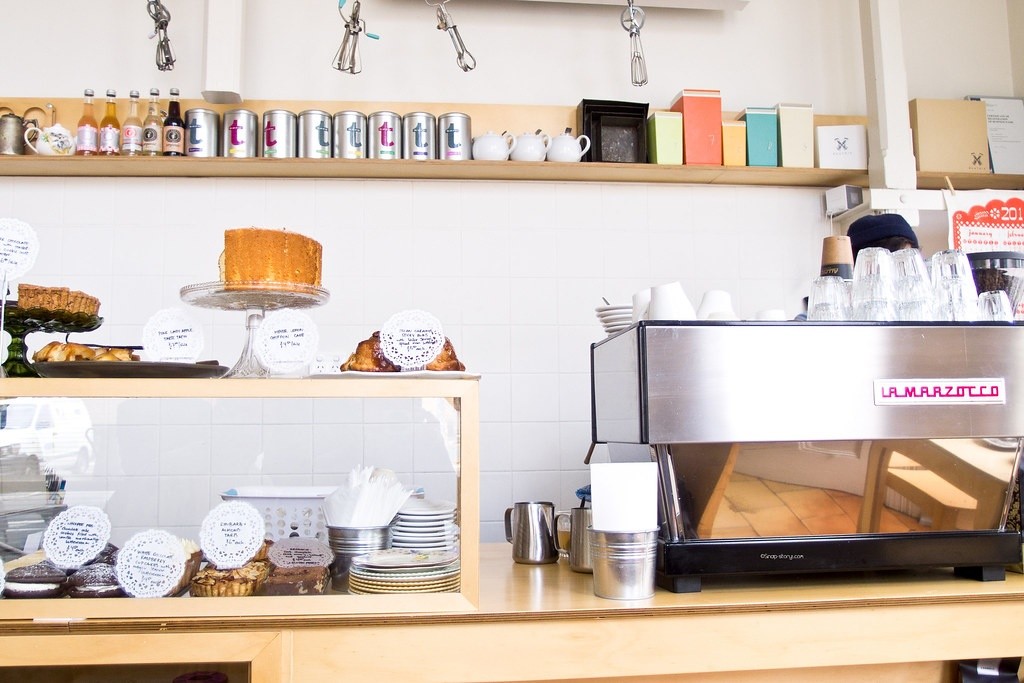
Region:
[472,131,517,161]
[24,122,77,156]
[547,133,590,163]
[512,133,552,161]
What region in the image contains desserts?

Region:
[172,534,332,595]
[1,540,134,598]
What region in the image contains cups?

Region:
[0,113,38,155]
[632,283,697,320]
[553,507,594,572]
[807,248,1013,321]
[585,525,661,600]
[324,524,393,592]
[820,236,855,281]
[504,502,559,564]
[697,290,740,320]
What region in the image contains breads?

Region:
[217,228,322,294]
[31,340,137,362]
[18,284,102,316]
[339,331,466,372]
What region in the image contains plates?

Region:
[303,371,482,380]
[595,304,632,337]
[347,498,461,593]
[33,360,229,378]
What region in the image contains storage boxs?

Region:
[576,87,814,169]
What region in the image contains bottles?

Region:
[185,109,218,157]
[162,87,185,156]
[223,109,258,158]
[368,111,401,159]
[299,110,332,158]
[123,91,142,156]
[142,89,164,157]
[438,112,471,160]
[78,89,98,156]
[99,90,120,156]
[263,109,297,158]
[403,111,436,160]
[333,110,367,158]
